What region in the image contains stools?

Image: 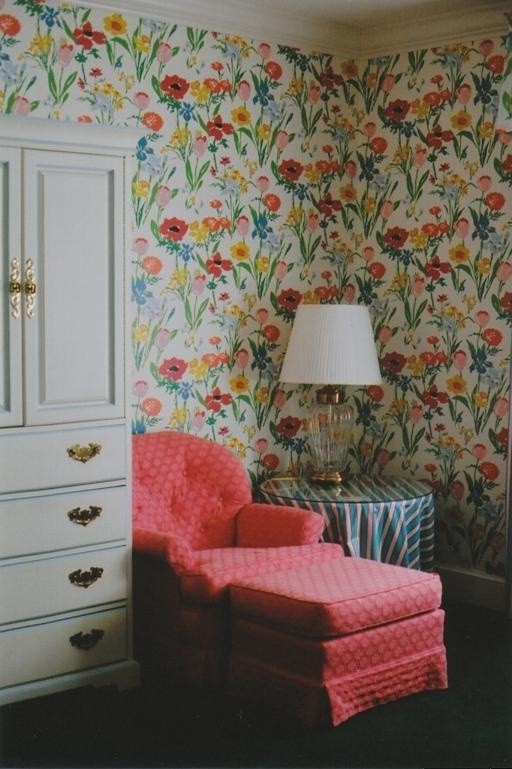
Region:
[227,555,448,729]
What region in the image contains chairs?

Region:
[132,430,326,687]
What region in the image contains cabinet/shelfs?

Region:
[0,423,132,708]
[0,112,143,428]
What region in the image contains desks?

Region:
[258,473,438,574]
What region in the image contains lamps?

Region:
[278,304,383,484]
